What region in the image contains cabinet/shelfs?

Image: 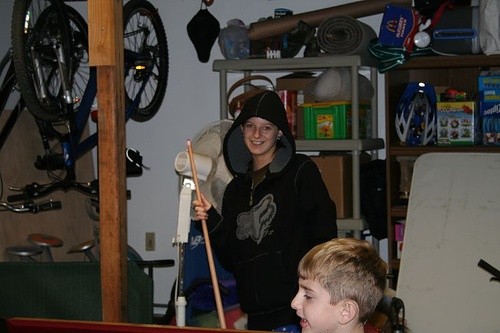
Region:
[209,53,380,255]
[381,53,500,287]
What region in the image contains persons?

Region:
[291,238,387,333]
[191,90,338,331]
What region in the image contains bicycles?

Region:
[0,0,169,215]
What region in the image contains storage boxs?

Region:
[478,76,500,146]
[434,101,481,147]
[308,155,354,219]
[301,100,373,140]
